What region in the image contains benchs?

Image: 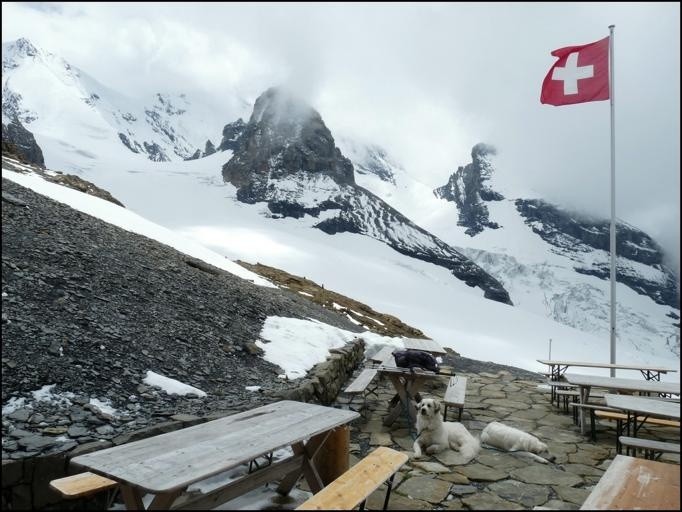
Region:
[540,35,610,106]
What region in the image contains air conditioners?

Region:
[413,398,482,459]
[480,422,556,465]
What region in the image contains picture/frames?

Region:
[68,397,361,510]
[377,336,448,426]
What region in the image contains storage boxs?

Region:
[344,346,468,424]
[535,357,682,512]
